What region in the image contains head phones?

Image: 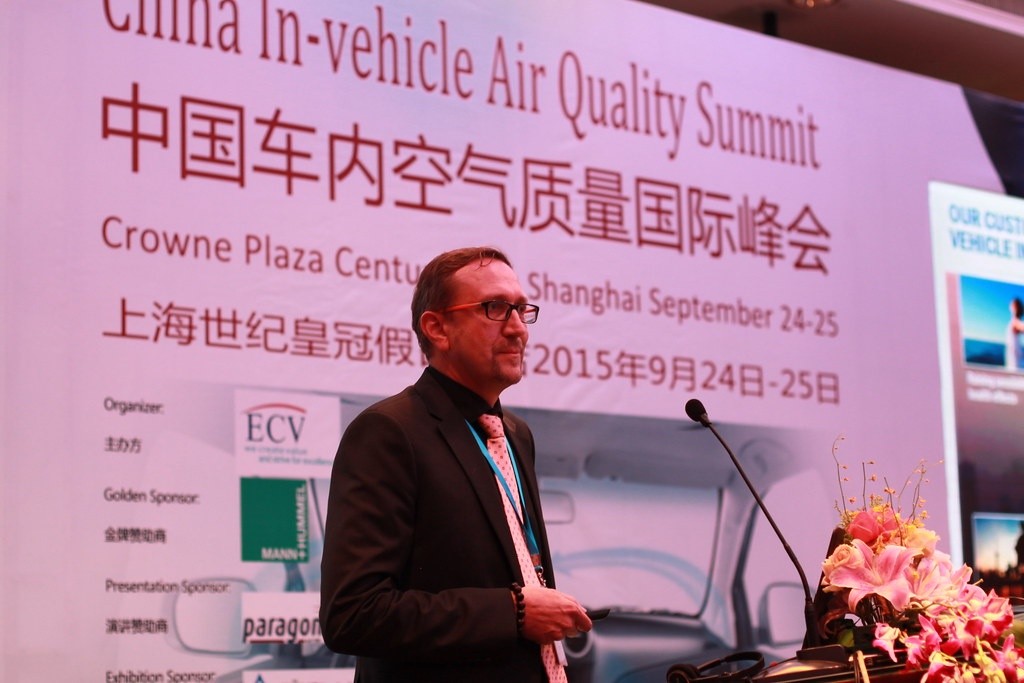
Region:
[666,650,765,683]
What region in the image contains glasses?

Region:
[435,299,540,324]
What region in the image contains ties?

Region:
[479,413,569,683]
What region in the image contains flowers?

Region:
[819,436,1024,683]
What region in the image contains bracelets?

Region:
[512,582,526,639]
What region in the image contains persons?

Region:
[319,248,593,683]
[1003,299,1024,369]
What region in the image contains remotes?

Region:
[586,608,612,621]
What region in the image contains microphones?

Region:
[684,398,819,646]
